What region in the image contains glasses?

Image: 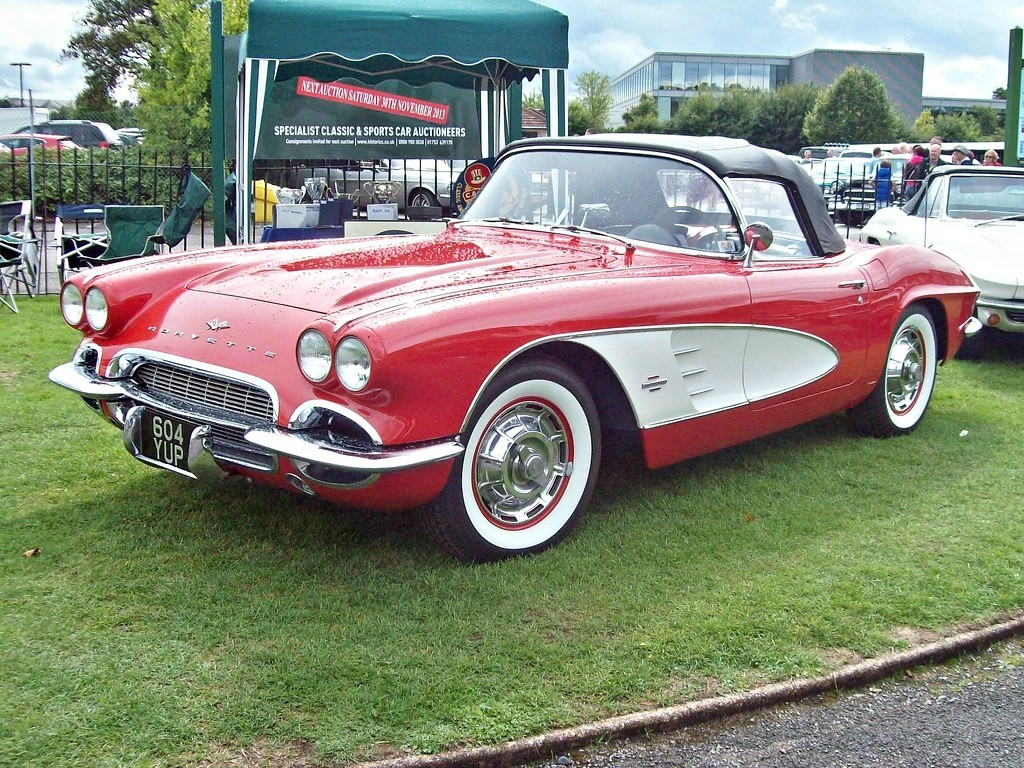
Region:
[986,155,994,159]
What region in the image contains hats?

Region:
[955,144,968,155]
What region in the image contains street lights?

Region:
[10,63,32,105]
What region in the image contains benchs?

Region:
[674,223,801,256]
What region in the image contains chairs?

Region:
[0,200,164,314]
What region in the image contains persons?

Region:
[568,128,601,226]
[827,149,836,158]
[916,137,946,193]
[981,149,1003,166]
[869,160,892,212]
[801,150,811,164]
[952,145,983,165]
[892,146,913,154]
[867,147,882,179]
[904,145,930,204]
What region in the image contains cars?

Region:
[857,164,1024,359]
[829,155,916,226]
[13,119,124,151]
[114,127,149,144]
[0,134,85,155]
[278,156,464,221]
[48,134,982,563]
[802,157,870,204]
[283,156,465,219]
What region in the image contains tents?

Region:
[236,0,569,246]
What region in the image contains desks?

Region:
[258,226,343,243]
